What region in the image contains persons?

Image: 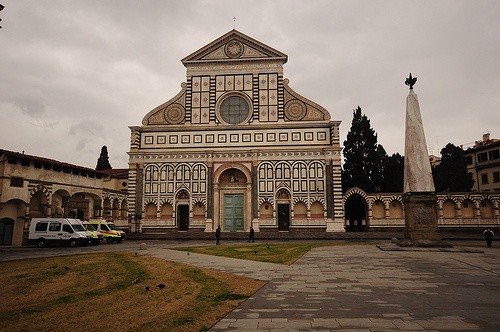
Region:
[248,227,255,242]
[483,226,495,248]
[215,225,221,245]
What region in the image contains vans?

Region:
[86,221,126,245]
[79,221,103,246]
[28,218,93,247]
